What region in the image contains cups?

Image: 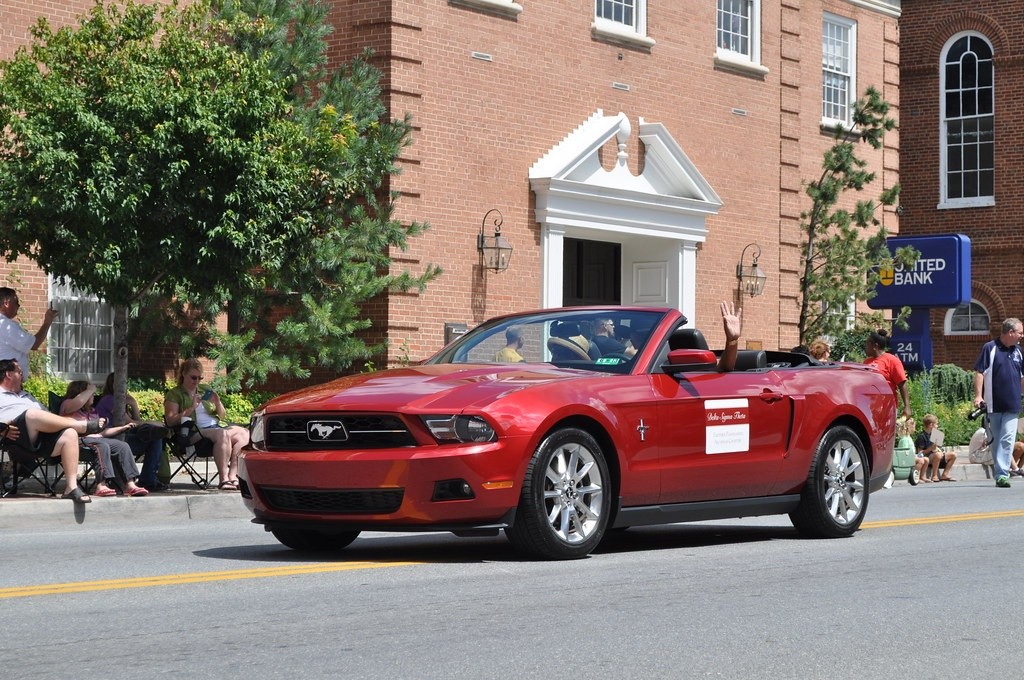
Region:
[180,416,192,436]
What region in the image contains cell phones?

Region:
[201,390,212,401]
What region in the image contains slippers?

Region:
[94,486,117,495]
[931,476,940,482]
[78,417,109,437]
[123,484,149,497]
[61,488,92,502]
[941,475,957,482]
[218,481,237,490]
[230,478,241,490]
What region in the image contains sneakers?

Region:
[996,473,1011,487]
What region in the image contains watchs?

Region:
[729,340,739,346]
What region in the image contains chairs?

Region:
[0,391,238,499]
[734,350,768,370]
[668,329,708,351]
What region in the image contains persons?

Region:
[494,325,526,364]
[0,358,109,503]
[898,416,932,485]
[969,416,1024,477]
[593,318,638,356]
[97,372,170,491]
[973,318,1024,487]
[58,381,149,496]
[0,287,59,382]
[718,300,742,371]
[790,342,831,361]
[914,414,956,482]
[863,329,911,489]
[165,358,249,489]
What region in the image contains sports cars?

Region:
[236,304,896,556]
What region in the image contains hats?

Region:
[630,314,657,332]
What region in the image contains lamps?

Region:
[478,208,513,274]
[736,244,767,301]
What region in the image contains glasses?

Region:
[184,370,204,380]
[602,324,613,326]
[7,368,23,374]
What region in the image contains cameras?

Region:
[968,401,987,421]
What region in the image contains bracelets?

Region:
[217,402,222,407]
[182,410,187,416]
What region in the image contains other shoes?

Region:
[138,477,170,491]
[884,477,893,488]
[1010,467,1024,476]
[922,478,931,482]
[919,478,924,484]
